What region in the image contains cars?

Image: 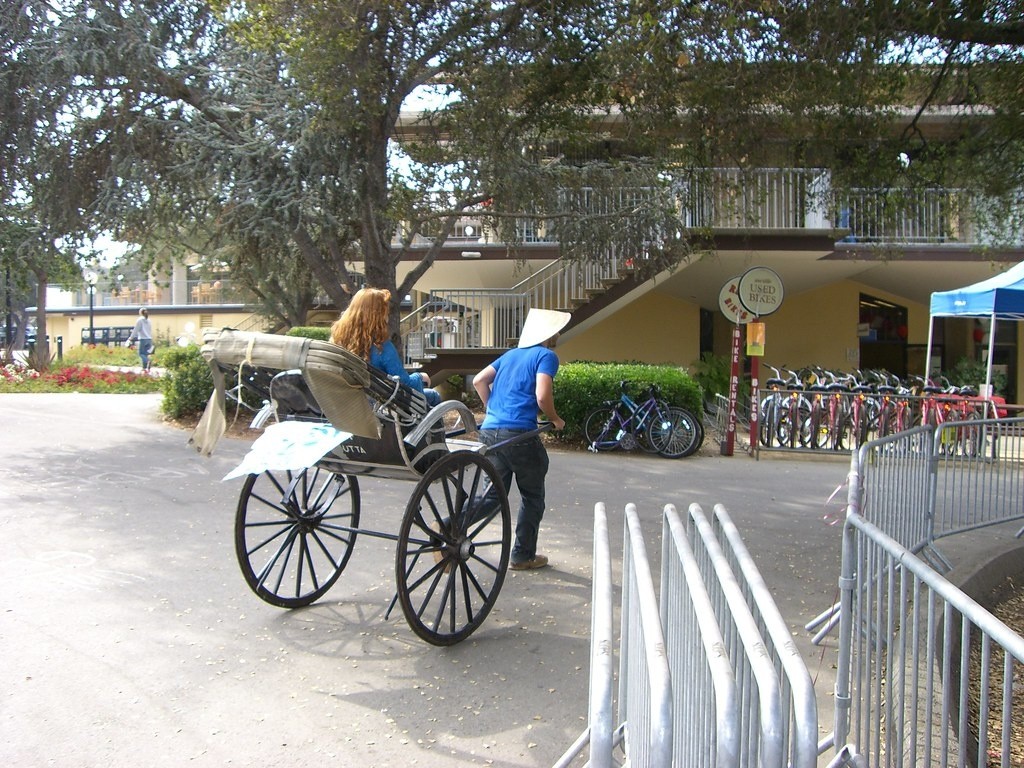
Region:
[0,326,37,350]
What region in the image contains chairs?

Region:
[198,325,450,475]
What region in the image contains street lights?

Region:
[85,272,98,346]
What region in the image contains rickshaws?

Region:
[197,326,567,649]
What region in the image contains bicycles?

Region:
[758,362,985,458]
[583,379,706,460]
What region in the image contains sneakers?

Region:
[509,554,548,569]
[431,521,450,572]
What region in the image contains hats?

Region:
[517,307,572,348]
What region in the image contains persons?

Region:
[128,307,154,373]
[429,305,573,571]
[329,289,443,419]
